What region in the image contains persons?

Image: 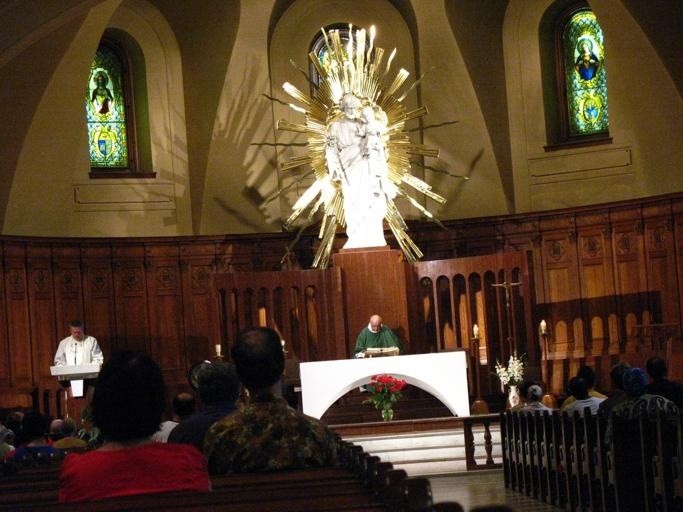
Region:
[168,359,242,442]
[607,368,679,447]
[7,409,63,458]
[319,89,405,249]
[149,392,197,443]
[57,350,212,502]
[562,368,608,407]
[599,364,631,414]
[643,357,683,403]
[562,376,602,415]
[519,386,551,415]
[51,420,85,447]
[9,411,25,438]
[355,315,404,358]
[54,321,104,364]
[570,34,607,85]
[202,328,346,474]
[87,64,115,119]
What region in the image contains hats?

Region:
[187,360,241,401]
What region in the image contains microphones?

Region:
[74,342,77,365]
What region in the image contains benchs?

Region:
[0,426,512,512]
